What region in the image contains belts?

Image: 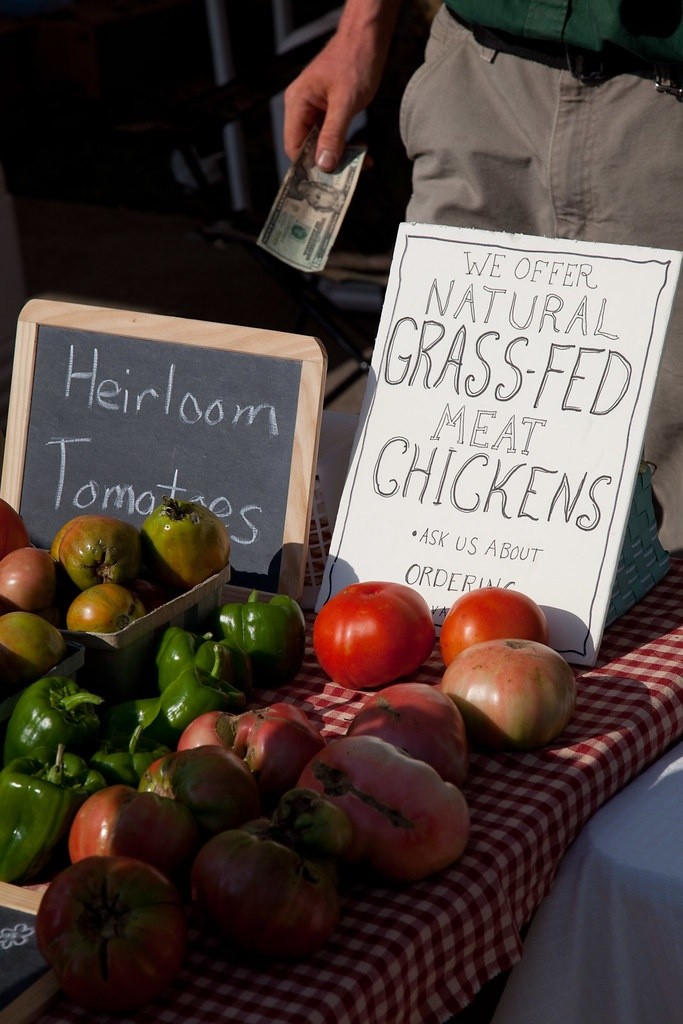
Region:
[444,6,648,81]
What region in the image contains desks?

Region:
[19,554,683,1023]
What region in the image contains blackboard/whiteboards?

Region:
[0,297,328,611]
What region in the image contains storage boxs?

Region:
[63,564,233,696]
[297,411,357,612]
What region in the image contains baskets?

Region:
[301,409,360,611]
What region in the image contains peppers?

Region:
[0,593,307,885]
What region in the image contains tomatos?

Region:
[0,492,231,701]
[34,582,576,1005]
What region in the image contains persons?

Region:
[284,0,682,557]
